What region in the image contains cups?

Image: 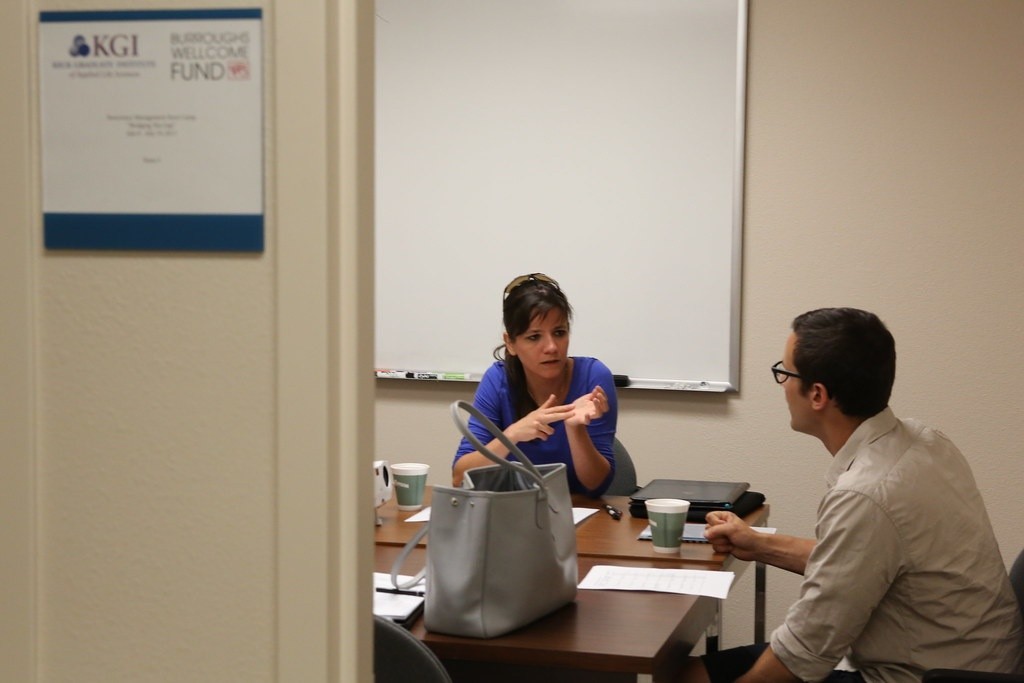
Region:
[645,499,691,554]
[390,463,430,512]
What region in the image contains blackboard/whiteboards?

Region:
[376,1,749,395]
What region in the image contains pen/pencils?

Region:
[603,505,622,519]
[377,588,425,597]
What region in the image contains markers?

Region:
[406,372,438,379]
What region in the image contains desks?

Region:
[373,482,769,683]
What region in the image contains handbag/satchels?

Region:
[390,400,580,637]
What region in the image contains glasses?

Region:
[500,273,559,310]
[772,361,811,384]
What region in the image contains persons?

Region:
[452,273,619,499]
[671,307,1024,683]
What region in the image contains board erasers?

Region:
[614,376,628,387]
